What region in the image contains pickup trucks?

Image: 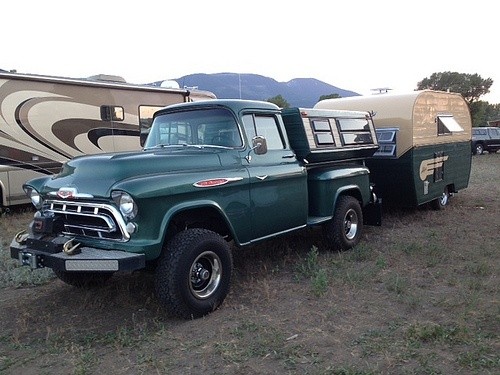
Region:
[9,90,380,319]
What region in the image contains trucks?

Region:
[0,68,218,216]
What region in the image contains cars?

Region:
[472,127,500,156]
